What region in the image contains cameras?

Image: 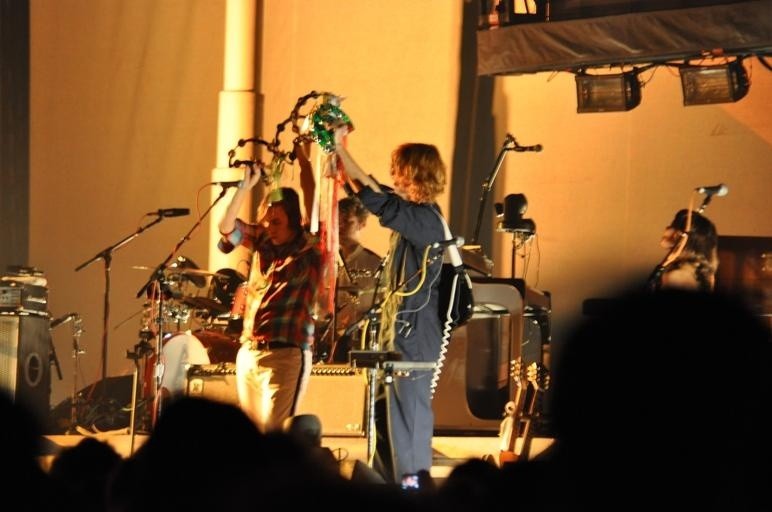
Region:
[493,193,535,233]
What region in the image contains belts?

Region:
[249,340,294,349]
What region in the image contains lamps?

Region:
[574,73,644,113]
[677,61,750,106]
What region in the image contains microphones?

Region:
[429,235,469,253]
[211,181,245,190]
[146,208,190,217]
[696,183,729,197]
[49,312,77,329]
[506,144,543,152]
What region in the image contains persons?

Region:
[314,196,385,364]
[645,205,721,294]
[216,130,327,432]
[311,113,451,488]
[1,280,772,512]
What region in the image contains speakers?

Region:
[0,315,50,432]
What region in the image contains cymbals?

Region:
[188,297,229,314]
[179,256,207,289]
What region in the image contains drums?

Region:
[143,331,239,430]
